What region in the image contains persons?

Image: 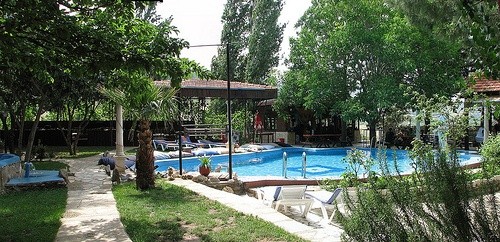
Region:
[181,136,206,148]
[384,127,396,149]
[395,129,406,149]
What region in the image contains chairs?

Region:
[303,187,345,224]
[262,182,315,220]
[152,134,227,151]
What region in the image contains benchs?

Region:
[301,141,313,147]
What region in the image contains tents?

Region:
[138,77,278,144]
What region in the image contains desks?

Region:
[305,134,342,148]
